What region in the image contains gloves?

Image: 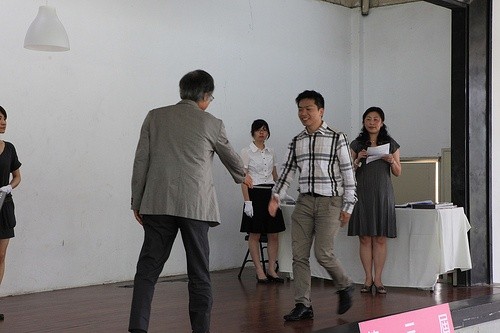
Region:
[244,201,253,218]
[0,185,13,194]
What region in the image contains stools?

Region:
[238,229,279,279]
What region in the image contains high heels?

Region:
[373,282,387,294]
[360,280,374,293]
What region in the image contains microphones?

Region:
[358,141,372,167]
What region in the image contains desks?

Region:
[275,203,474,295]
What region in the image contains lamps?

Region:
[23,0,73,53]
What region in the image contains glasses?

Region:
[256,129,267,133]
[210,94,214,101]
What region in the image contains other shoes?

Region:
[267,274,284,282]
[256,275,269,282]
[0,314,4,320]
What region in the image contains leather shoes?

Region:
[337,283,354,314]
[283,303,313,320]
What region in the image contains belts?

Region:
[253,186,272,188]
[306,192,326,197]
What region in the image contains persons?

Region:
[347,107,401,294]
[268,90,358,321]
[239,119,286,285]
[128,70,254,333]
[0,106,22,321]
